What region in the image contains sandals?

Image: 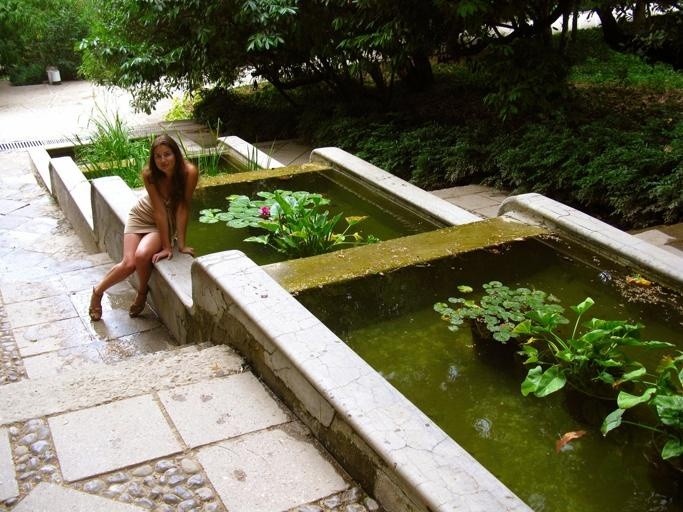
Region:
[88,286,104,322]
[129,286,151,318]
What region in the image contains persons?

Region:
[89,134,198,321]
